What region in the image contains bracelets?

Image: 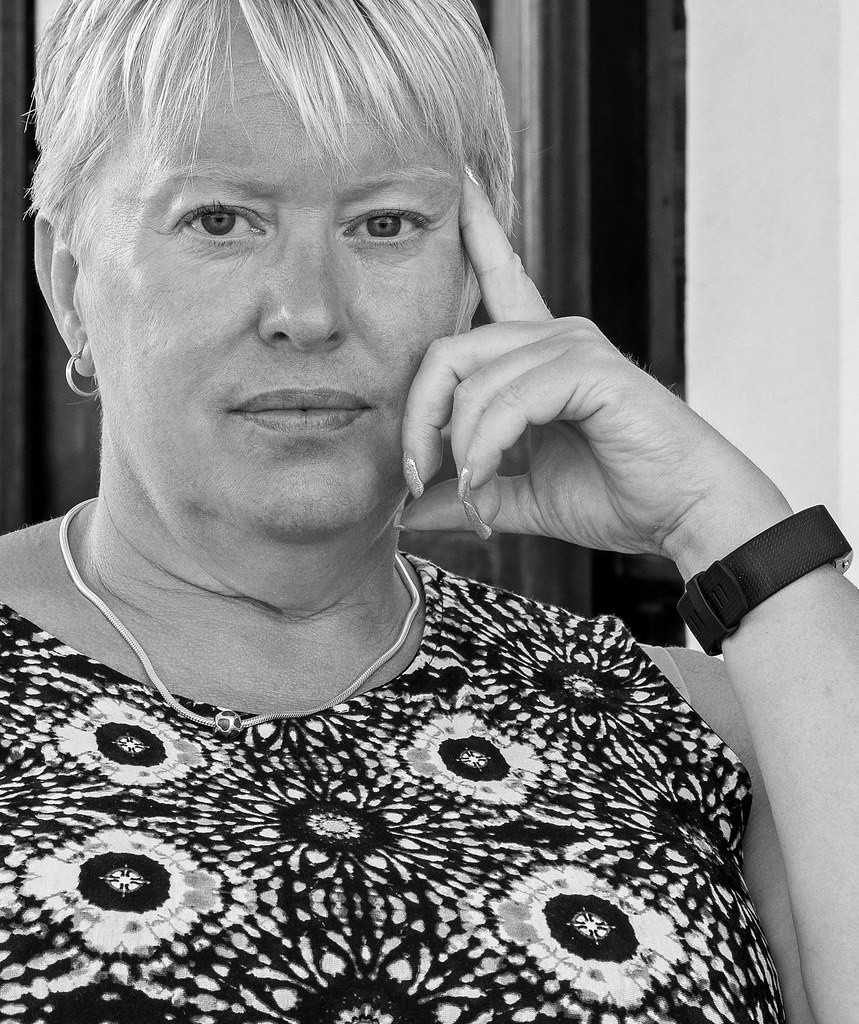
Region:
[678,502,852,656]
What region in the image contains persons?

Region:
[1,0,856,1022]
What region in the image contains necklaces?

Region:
[58,495,424,735]
